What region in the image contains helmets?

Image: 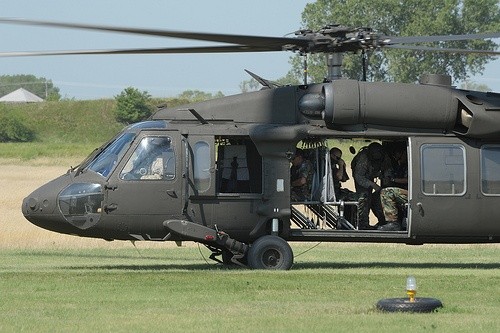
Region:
[149,137,170,156]
[368,142,384,162]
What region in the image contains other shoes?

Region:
[378,222,402,231]
[360,225,377,230]
[341,222,356,229]
[374,221,387,228]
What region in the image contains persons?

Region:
[290,148,313,201]
[350,141,384,230]
[377,146,409,230]
[370,150,396,228]
[330,147,363,230]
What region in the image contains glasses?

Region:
[331,153,341,161]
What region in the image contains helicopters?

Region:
[1,17,500,270]
